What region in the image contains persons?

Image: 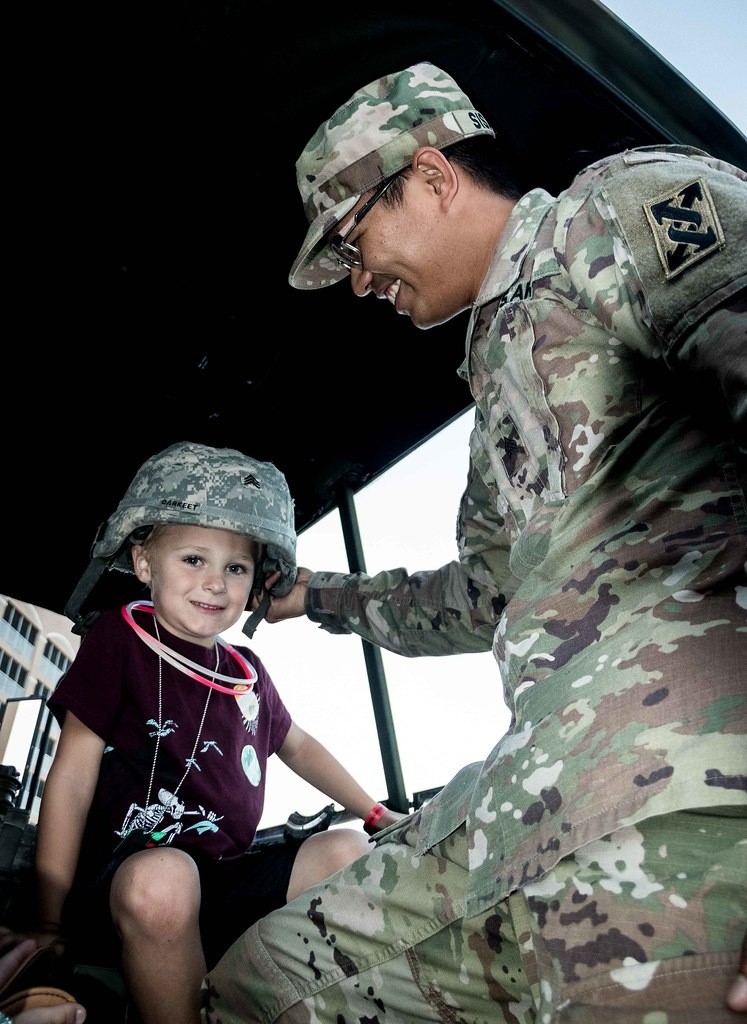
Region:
[2,442,412,1024]
[194,61,747,1024]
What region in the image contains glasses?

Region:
[330,168,406,272]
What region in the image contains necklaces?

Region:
[121,600,258,834]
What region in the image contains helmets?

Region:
[93,441,297,602]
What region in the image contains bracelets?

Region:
[363,803,388,830]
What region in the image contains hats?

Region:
[288,60,498,291]
